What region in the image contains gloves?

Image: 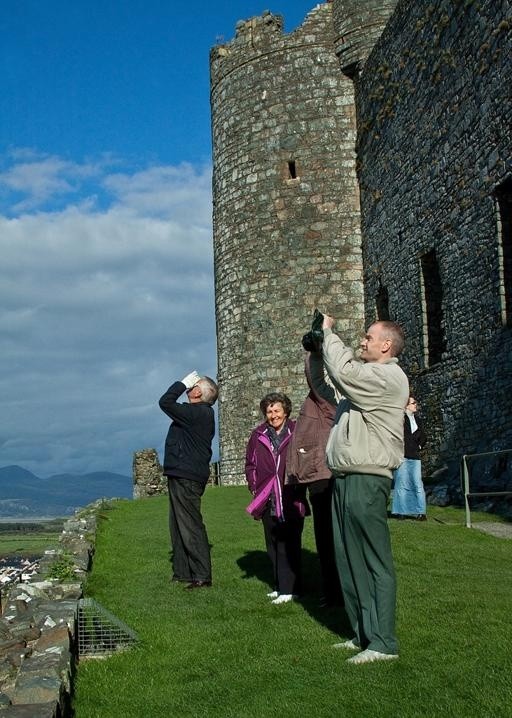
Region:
[181,371,201,389]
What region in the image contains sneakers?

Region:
[344,648,400,665]
[266,590,294,605]
[332,639,365,651]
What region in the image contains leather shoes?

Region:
[183,576,212,590]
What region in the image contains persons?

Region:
[308,314,410,665]
[245,393,311,605]
[391,395,427,521]
[158,370,218,591]
[286,351,342,615]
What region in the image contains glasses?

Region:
[408,400,416,405]
